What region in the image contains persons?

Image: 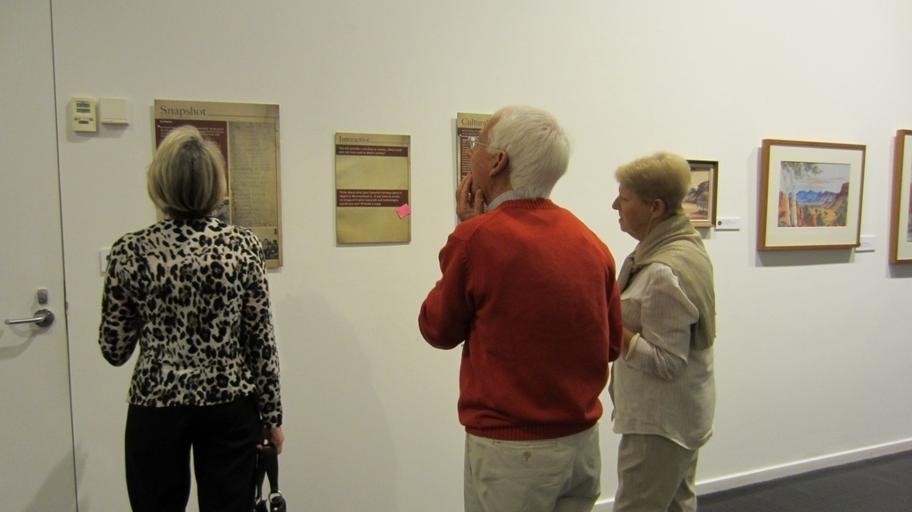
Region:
[606,150,718,511]
[418,105,623,511]
[97,123,284,512]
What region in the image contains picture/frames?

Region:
[680,156,722,232]
[756,137,868,253]
[885,126,912,267]
